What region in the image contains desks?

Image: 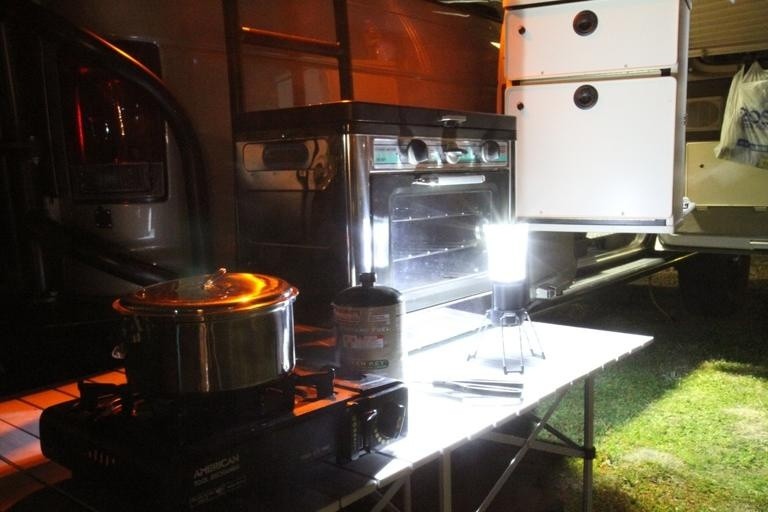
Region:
[0,311,657,511]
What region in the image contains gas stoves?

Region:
[40,369,409,499]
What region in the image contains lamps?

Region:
[465,217,550,377]
[408,172,486,188]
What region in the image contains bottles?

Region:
[331,270,404,378]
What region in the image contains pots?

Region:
[110,267,303,400]
